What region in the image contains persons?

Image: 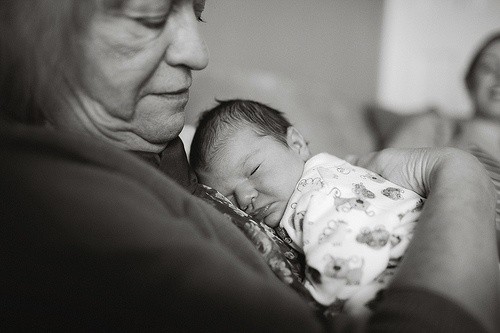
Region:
[384,31,499,260]
[190,99,439,332]
[0,0,499,332]
